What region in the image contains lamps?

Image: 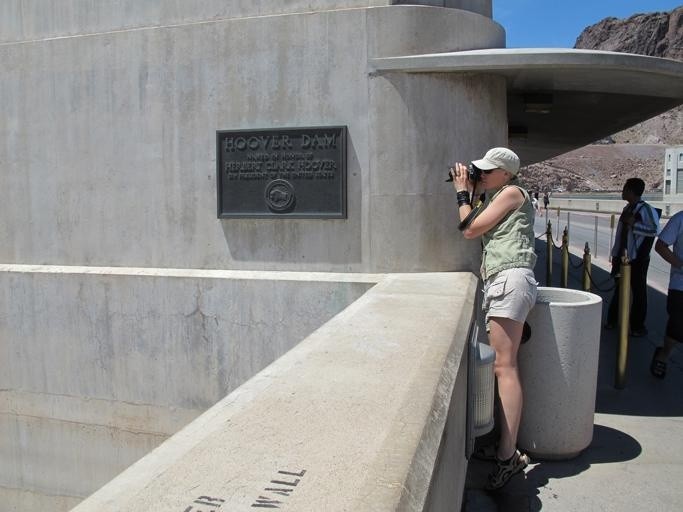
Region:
[464,319,497,464]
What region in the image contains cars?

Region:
[598,136,616,144]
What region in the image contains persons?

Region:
[603,178,657,337]
[535,189,538,209]
[650,210,683,379]
[544,191,549,209]
[450,146,540,495]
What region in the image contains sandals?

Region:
[484,450,531,490]
[474,443,498,460]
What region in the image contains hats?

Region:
[470,146,520,175]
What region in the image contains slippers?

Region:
[651,346,667,378]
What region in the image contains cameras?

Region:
[445,164,482,183]
